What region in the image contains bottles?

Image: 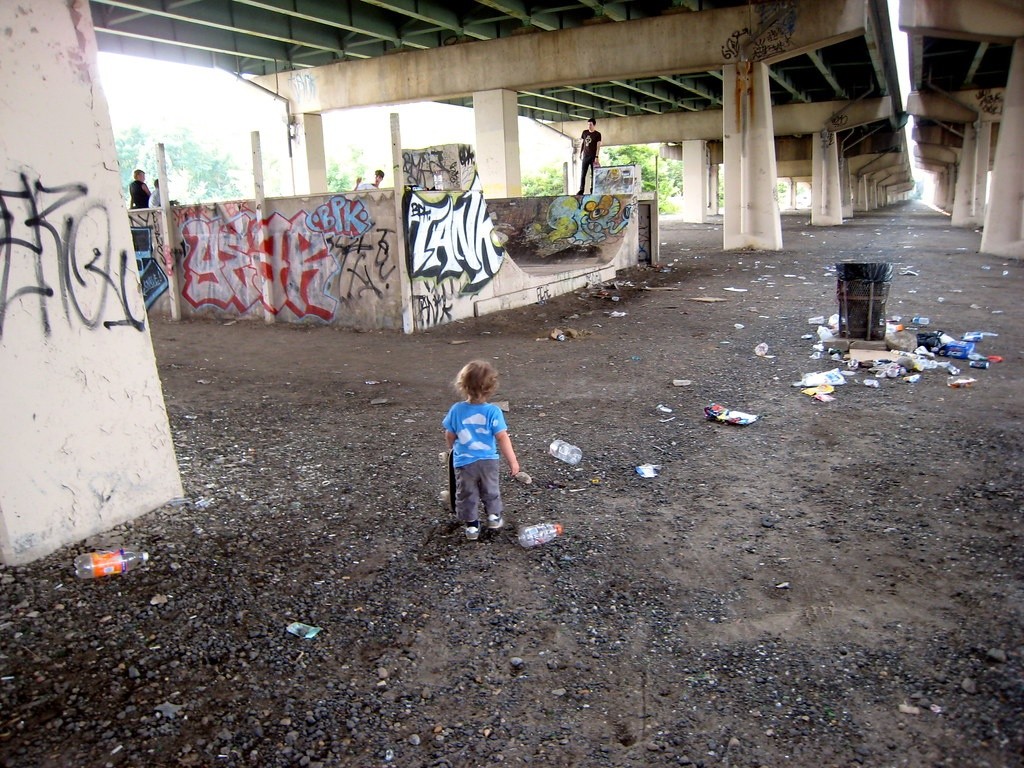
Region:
[755,343,768,356]
[550,440,582,465]
[73,547,148,579]
[910,318,929,325]
[518,523,563,548]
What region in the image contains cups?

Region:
[808,316,824,325]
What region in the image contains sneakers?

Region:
[487,516,504,529]
[466,521,481,540]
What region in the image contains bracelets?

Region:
[595,156,598,158]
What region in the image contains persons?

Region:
[575,118,602,195]
[129,169,152,209]
[352,169,384,192]
[148,179,180,208]
[441,359,520,541]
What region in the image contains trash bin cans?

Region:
[834,261,895,340]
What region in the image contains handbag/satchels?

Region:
[130,199,136,209]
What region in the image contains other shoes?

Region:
[591,191,592,195]
[576,191,584,195]
